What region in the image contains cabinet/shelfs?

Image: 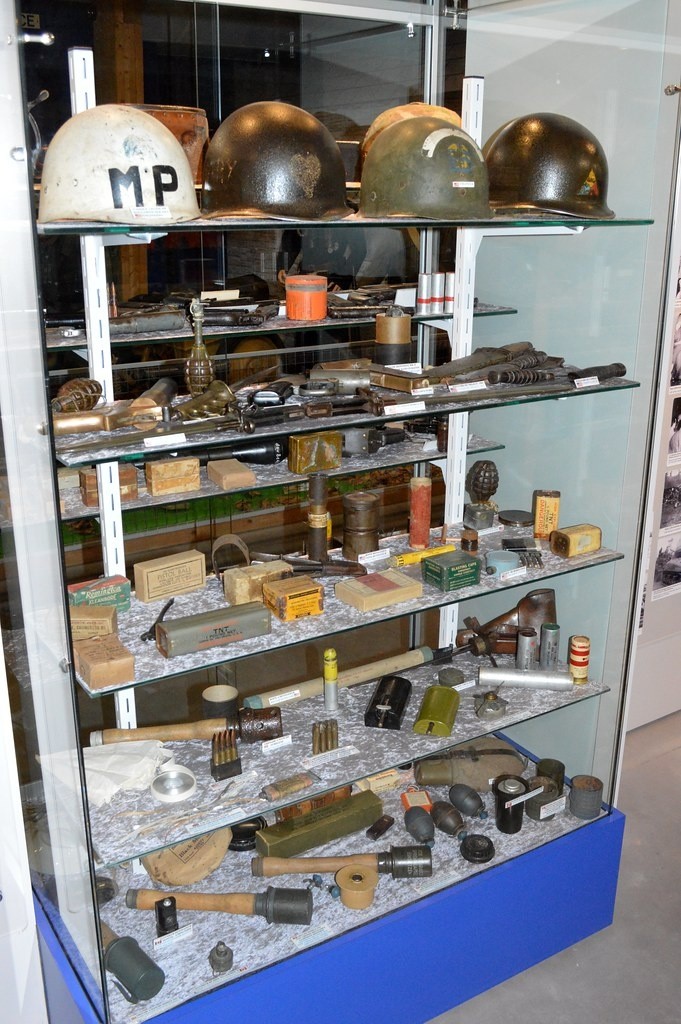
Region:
[3,0,676,1022]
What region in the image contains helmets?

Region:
[361,102,461,153]
[483,111,614,220]
[35,105,202,224]
[358,116,494,221]
[201,100,355,221]
[224,340,282,392]
